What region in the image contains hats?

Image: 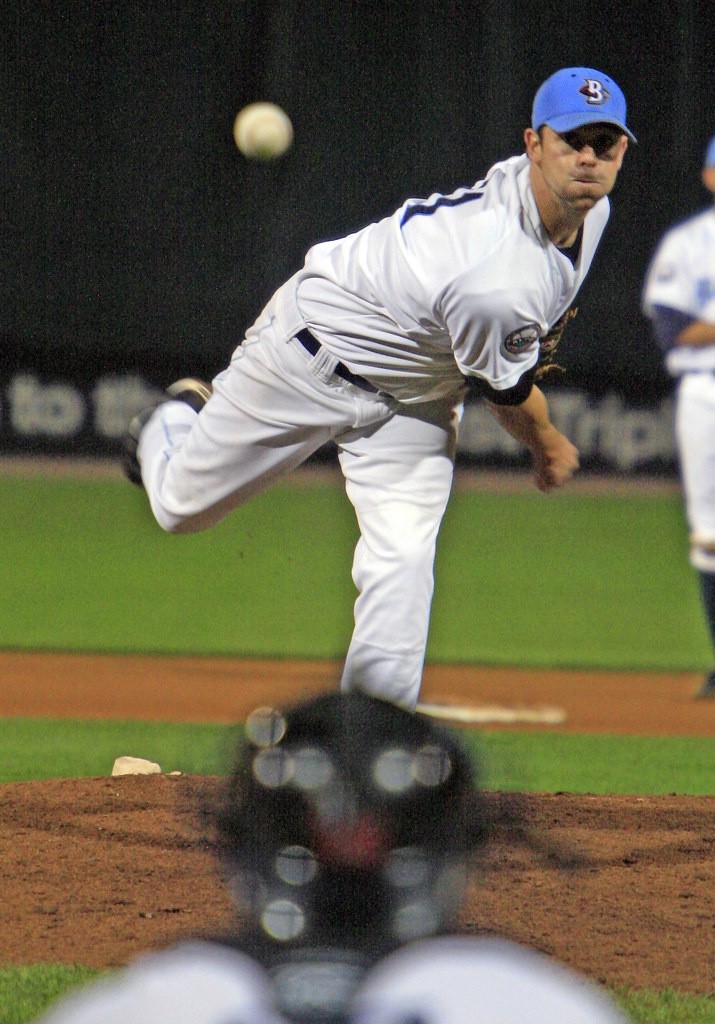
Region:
[703,139,715,167]
[531,66,639,145]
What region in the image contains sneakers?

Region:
[121,377,212,487]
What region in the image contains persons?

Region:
[642,140,715,697]
[32,691,628,1024]
[125,69,635,711]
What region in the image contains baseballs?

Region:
[232,101,295,161]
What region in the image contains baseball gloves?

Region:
[530,304,580,389]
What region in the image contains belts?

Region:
[295,328,390,400]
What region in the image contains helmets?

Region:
[213,691,470,949]
